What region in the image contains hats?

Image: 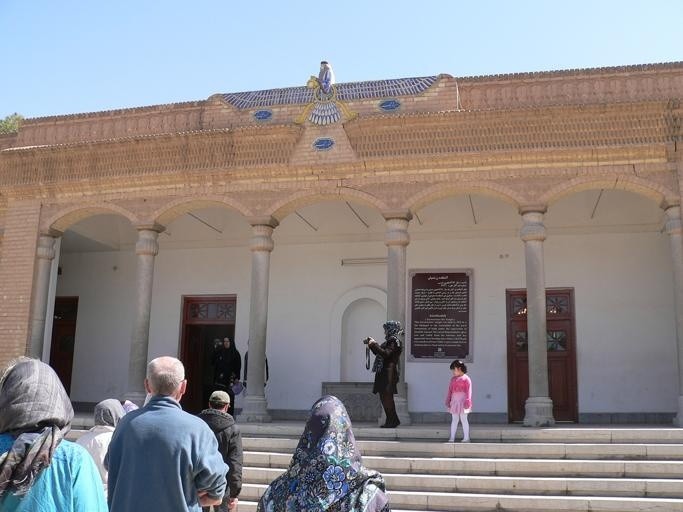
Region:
[208,390,231,408]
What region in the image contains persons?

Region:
[210,335,268,419]
[445,359,473,443]
[195,390,244,512]
[370,320,404,429]
[75,395,126,501]
[256,394,391,512]
[100,354,231,512]
[119,399,139,413]
[0,356,108,512]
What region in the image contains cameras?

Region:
[363,337,371,344]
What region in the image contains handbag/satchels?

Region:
[231,382,243,394]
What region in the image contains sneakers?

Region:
[379,417,400,428]
